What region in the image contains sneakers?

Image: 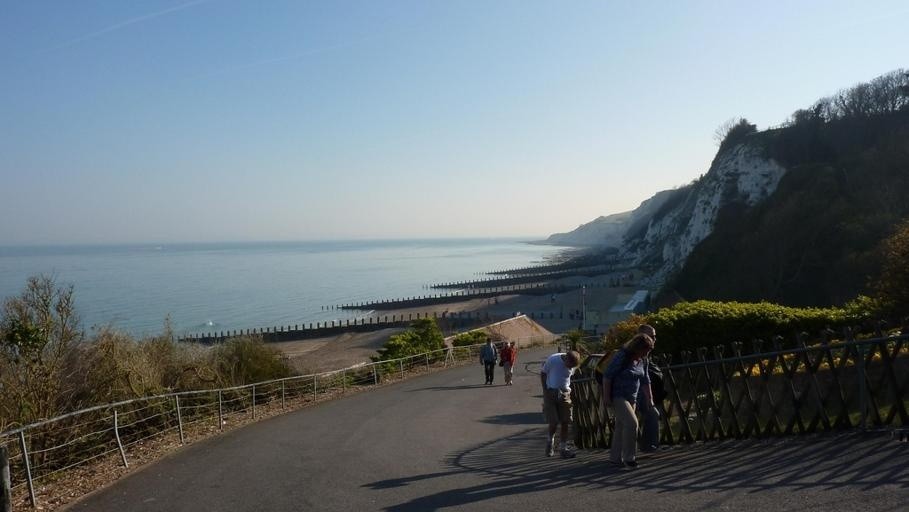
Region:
[608,459,637,469]
[545,441,555,456]
[485,381,492,385]
[505,381,512,385]
[559,450,575,458]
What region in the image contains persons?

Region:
[602,332,655,468]
[637,323,663,453]
[500,340,515,385]
[479,337,498,385]
[540,350,581,458]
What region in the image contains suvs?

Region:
[573,354,665,411]
